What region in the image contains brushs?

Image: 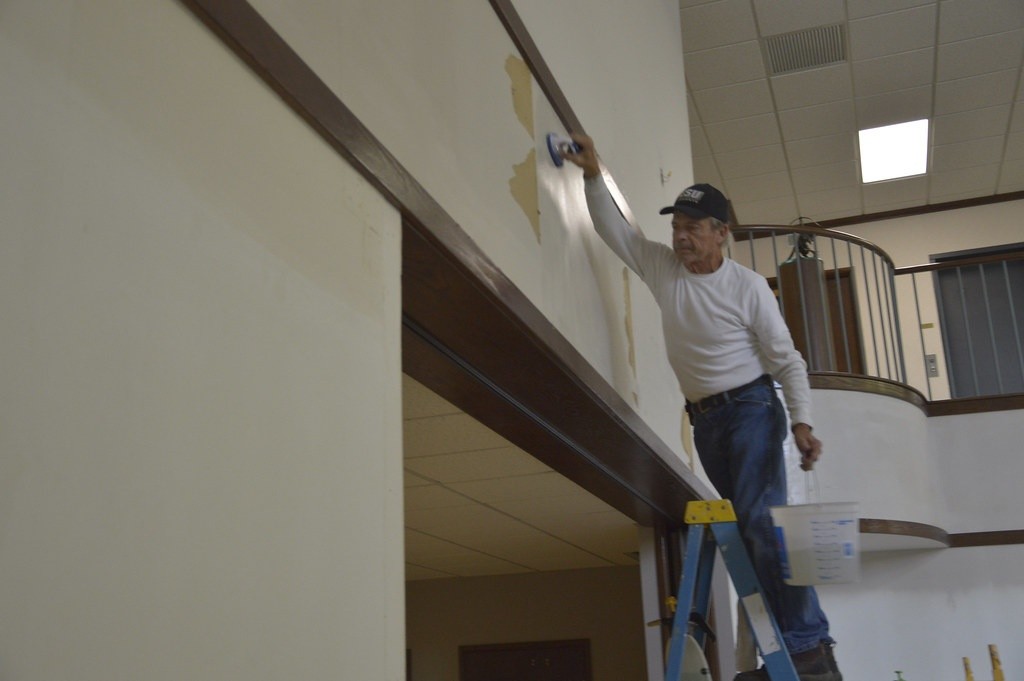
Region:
[546,132,581,167]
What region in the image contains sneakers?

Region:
[734,641,843,681]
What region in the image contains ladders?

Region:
[664,499,800,681]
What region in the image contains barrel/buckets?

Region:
[770,455,861,586]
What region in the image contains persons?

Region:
[561,133,843,681]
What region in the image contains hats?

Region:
[659,183,728,225]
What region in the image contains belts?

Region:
[691,374,773,416]
